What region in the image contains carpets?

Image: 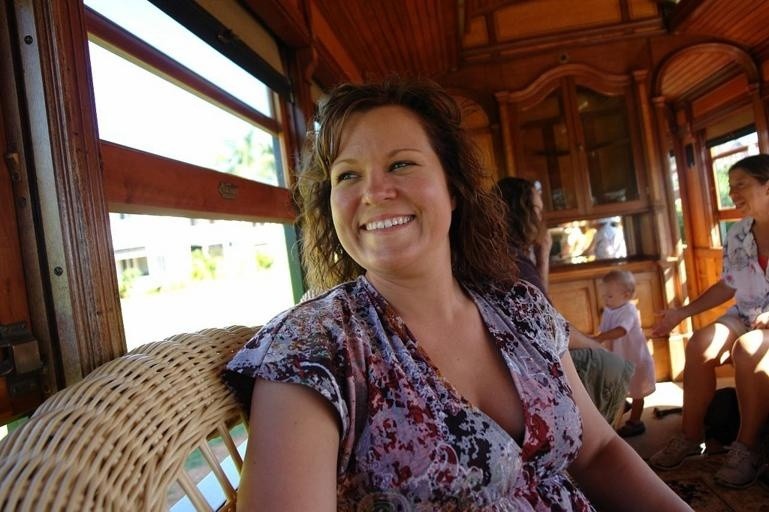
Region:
[643,449,768,512]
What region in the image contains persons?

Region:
[653,154,769,490]
[586,267,656,436]
[224,68,695,512]
[581,213,628,263]
[487,175,635,433]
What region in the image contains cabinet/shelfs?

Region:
[494,60,656,226]
[546,256,671,383]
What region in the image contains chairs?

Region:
[0,323,275,512]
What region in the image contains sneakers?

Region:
[621,399,632,416]
[616,420,645,438]
[650,432,707,471]
[713,441,761,490]
